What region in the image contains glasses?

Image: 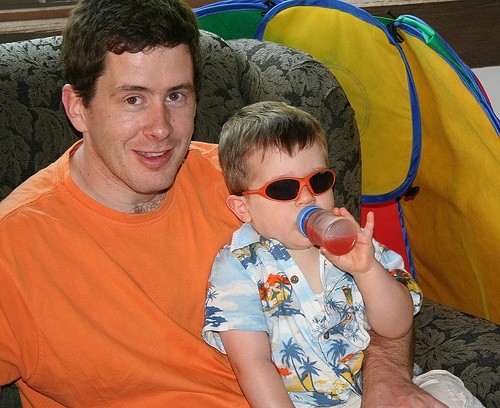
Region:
[241,167,336,202]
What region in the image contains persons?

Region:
[202,102,486,408]
[0,0,451,408]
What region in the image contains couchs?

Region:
[0,29,500,408]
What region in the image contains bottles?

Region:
[296,205,357,256]
